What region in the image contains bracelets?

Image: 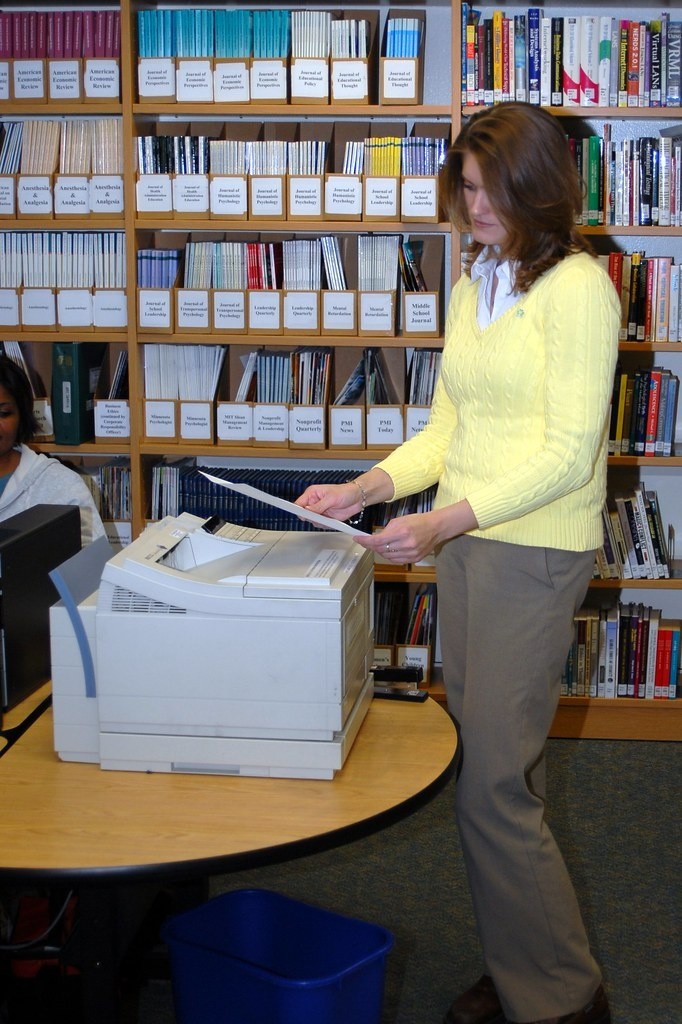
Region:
[345,480,367,524]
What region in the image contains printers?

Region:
[48,513,374,782]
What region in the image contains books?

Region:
[0,4,681,701]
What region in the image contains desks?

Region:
[0,676,464,1024]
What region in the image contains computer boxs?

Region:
[0,502,82,714]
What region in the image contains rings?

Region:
[387,543,390,553]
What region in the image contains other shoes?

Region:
[443,974,506,1024]
[506,984,610,1024]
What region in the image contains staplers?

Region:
[369,665,429,703]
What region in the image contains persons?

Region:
[294,102,620,1024]
[0,355,109,548]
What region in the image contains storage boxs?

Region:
[168,886,394,1024]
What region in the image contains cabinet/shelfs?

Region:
[121,0,461,704]
[2,0,133,556]
[457,0,682,742]
